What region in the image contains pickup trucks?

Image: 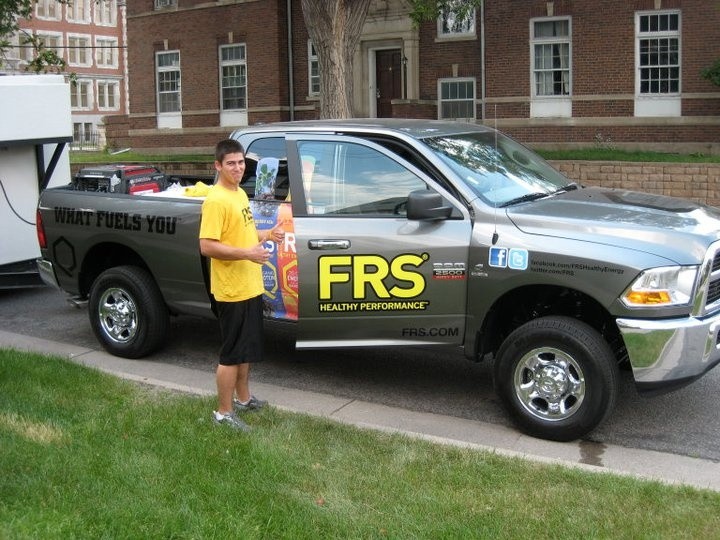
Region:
[34,115,720,443]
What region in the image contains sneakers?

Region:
[212,410,251,433]
[233,392,268,412]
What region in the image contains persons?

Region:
[200,139,285,433]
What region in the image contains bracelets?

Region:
[269,229,272,239]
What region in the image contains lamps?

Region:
[402,54,408,65]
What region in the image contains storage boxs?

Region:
[73,162,168,196]
